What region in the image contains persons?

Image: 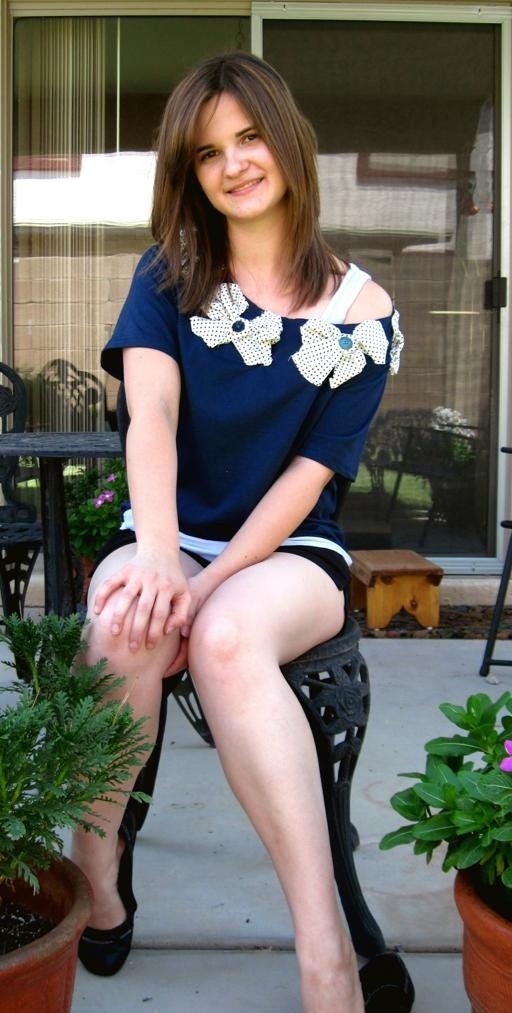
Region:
[55,52,402,1011]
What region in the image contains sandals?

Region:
[78,808,137,976]
[359,952,415,1013]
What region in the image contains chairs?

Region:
[385,418,489,546]
[0,361,126,621]
[33,358,107,431]
[111,381,419,1013]
[479,446,512,678]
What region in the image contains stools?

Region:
[346,547,446,631]
[0,519,45,630]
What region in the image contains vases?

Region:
[452,867,512,1013]
[77,552,105,605]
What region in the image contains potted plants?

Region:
[1,602,147,1013]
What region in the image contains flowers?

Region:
[67,458,131,557]
[375,689,512,912]
